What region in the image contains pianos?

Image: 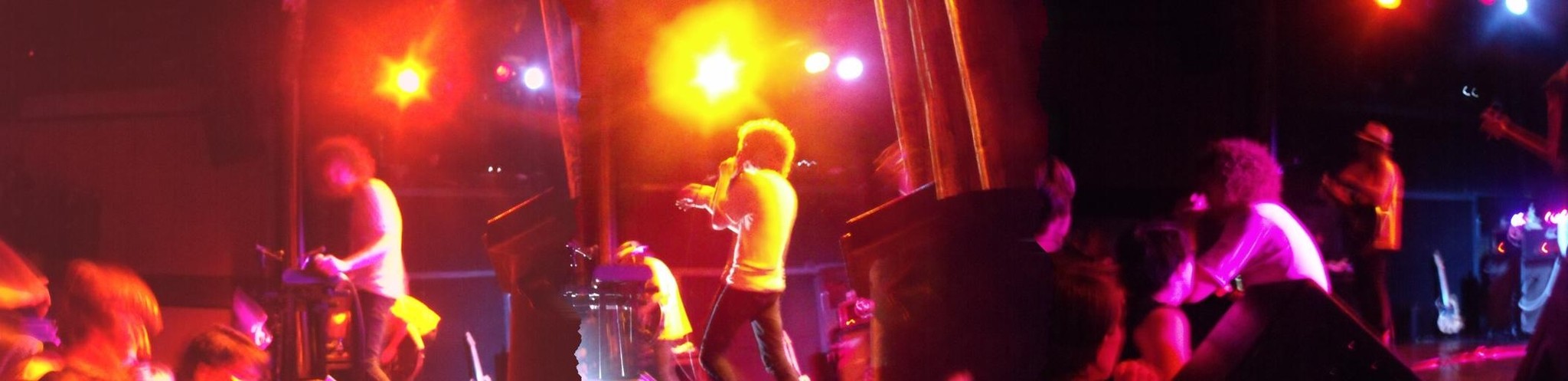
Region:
[592,264,652,293]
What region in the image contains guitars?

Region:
[1432,251,1464,336]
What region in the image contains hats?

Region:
[1355,123,1394,149]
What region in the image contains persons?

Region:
[675,117,804,379]
[301,134,406,381]
[1029,64,1567,381]
[0,239,265,381]
[378,291,445,380]
[612,237,695,380]
